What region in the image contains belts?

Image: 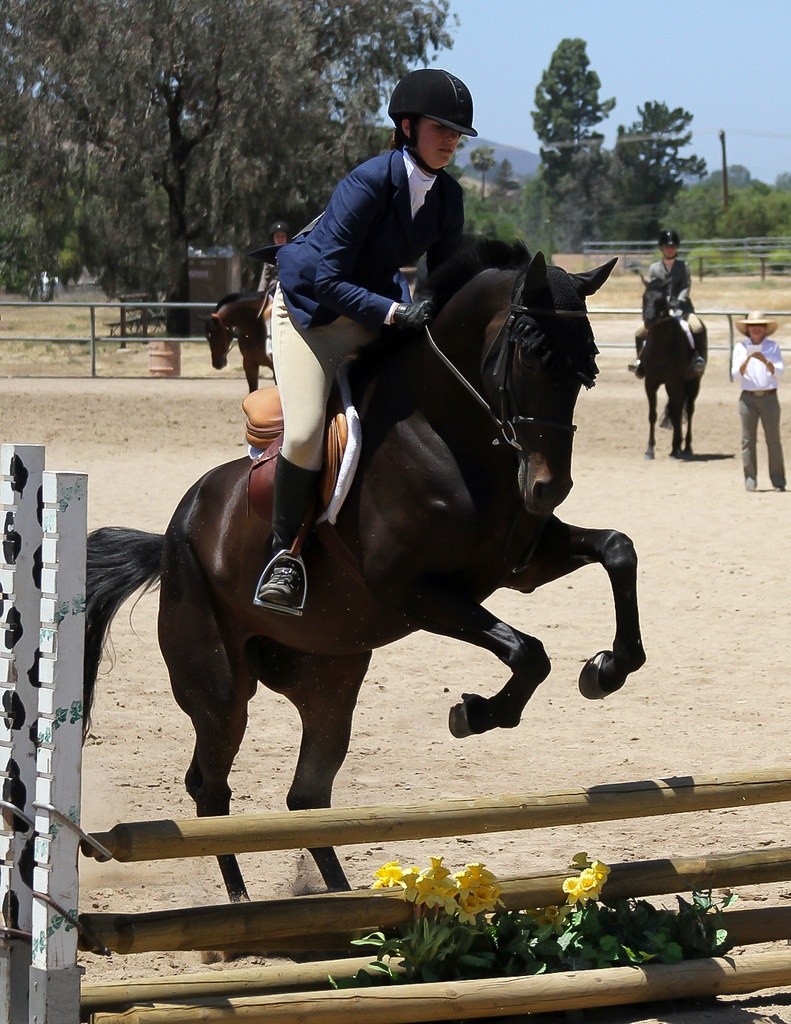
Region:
[745,389,775,397]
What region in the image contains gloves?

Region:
[393,300,434,329]
[676,301,684,309]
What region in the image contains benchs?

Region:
[109,302,165,337]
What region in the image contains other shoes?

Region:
[776,486,784,491]
[746,477,755,489]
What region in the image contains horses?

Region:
[84,234,708,902]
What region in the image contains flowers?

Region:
[326,857,735,992]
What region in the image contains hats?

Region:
[736,311,778,337]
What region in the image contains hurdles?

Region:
[2,442,791,1023]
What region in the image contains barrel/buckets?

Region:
[150,341,182,376]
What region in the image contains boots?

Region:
[689,327,708,379]
[627,336,645,368]
[258,447,325,606]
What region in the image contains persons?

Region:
[257,69,478,606]
[628,230,705,376]
[258,222,289,292]
[731,312,787,492]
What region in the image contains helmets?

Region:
[658,230,680,247]
[269,221,289,234]
[388,69,478,137]
